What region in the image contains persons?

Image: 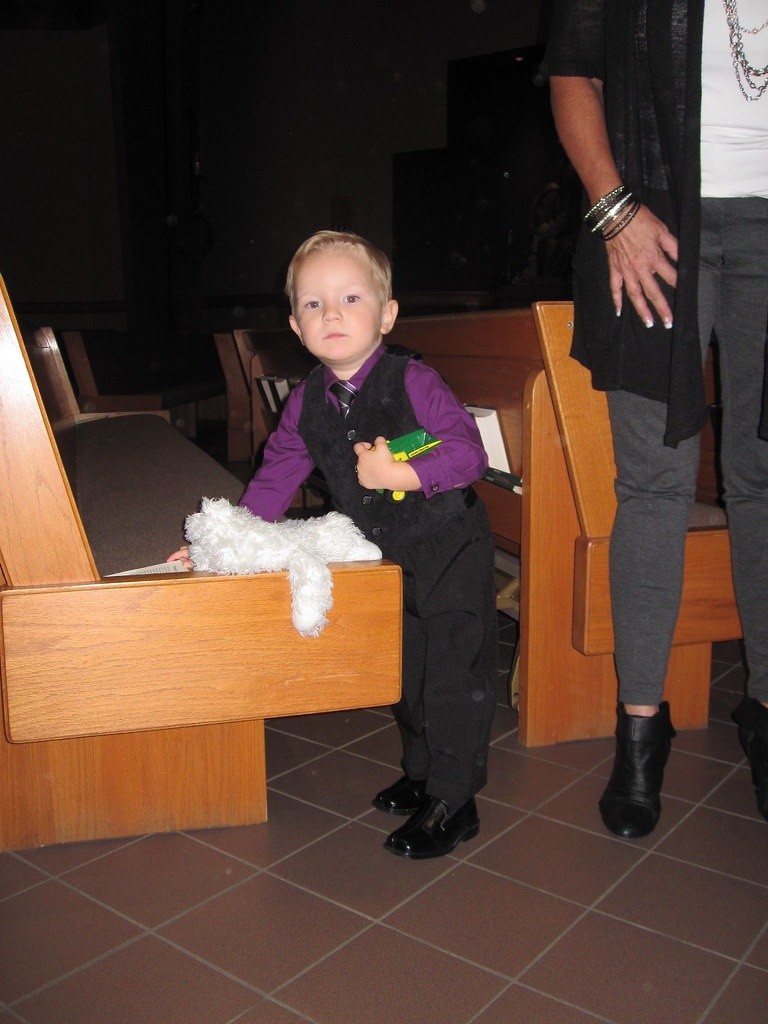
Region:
[167,229,500,860]
[541,0,768,840]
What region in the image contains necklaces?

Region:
[723,0,768,102]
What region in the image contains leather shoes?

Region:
[383,795,480,859]
[598,701,677,838]
[372,775,433,815]
[730,697,768,821]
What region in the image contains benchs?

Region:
[143,332,252,462]
[24,326,172,430]
[231,300,743,749]
[0,273,405,850]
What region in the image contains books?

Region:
[255,374,512,475]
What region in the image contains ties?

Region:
[330,380,359,419]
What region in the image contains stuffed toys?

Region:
[183,495,382,638]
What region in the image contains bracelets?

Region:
[584,185,641,242]
